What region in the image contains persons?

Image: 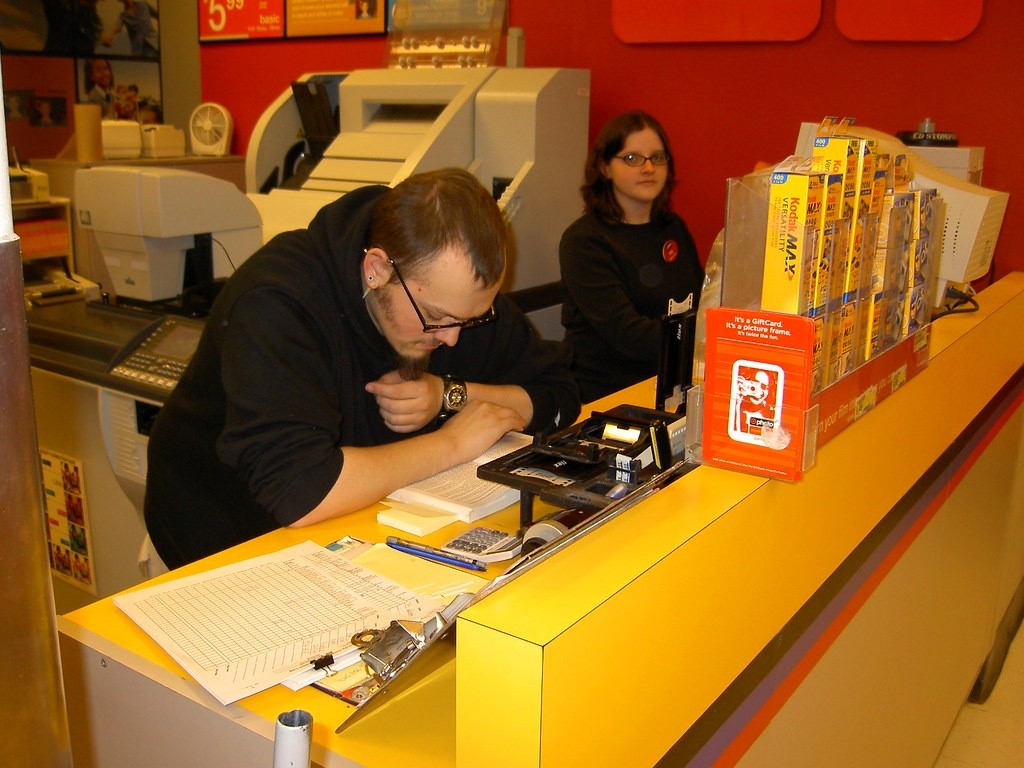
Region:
[0,0,163,124]
[357,0,373,20]
[141,164,583,571]
[557,108,704,405]
[54,462,91,585]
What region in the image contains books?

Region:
[377,428,533,538]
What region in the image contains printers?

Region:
[72,167,262,300]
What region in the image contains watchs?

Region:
[439,374,467,424]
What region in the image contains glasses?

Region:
[612,152,670,167]
[363,248,497,334]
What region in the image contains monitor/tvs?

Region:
[794,123,1011,310]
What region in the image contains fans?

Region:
[189,103,233,156]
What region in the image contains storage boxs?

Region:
[759,115,939,398]
[101,119,143,160]
[141,125,185,159]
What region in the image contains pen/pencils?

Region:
[386,536,488,573]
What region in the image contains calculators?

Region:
[439,526,525,565]
